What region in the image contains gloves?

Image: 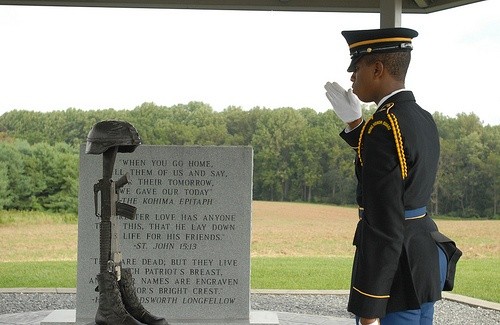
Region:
[324,81,363,123]
[358,317,379,325]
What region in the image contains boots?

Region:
[117,267,168,325]
[95,272,146,325]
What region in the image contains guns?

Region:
[93,149,136,274]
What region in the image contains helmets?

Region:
[85,120,140,155]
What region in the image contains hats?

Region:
[341,28,418,72]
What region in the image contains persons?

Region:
[324,28,463,325]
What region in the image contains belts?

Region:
[358,206,428,221]
